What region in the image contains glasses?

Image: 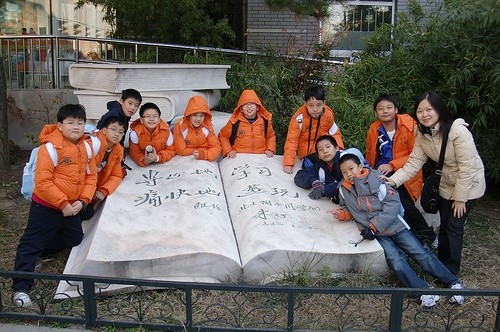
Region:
[107,127,125,136]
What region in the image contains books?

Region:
[68,62,233,147]
[53,148,390,300]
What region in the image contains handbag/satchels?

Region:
[420,175,439,215]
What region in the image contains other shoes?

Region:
[420,287,440,308]
[13,291,32,308]
[449,284,465,304]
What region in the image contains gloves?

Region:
[360,228,376,241]
[308,181,325,200]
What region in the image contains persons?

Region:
[381,90,487,287]
[12,89,142,307]
[293,93,438,249]
[128,103,176,167]
[173,96,222,161]
[218,90,277,158]
[326,154,465,307]
[282,86,345,173]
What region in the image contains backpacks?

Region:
[19,143,57,200]
